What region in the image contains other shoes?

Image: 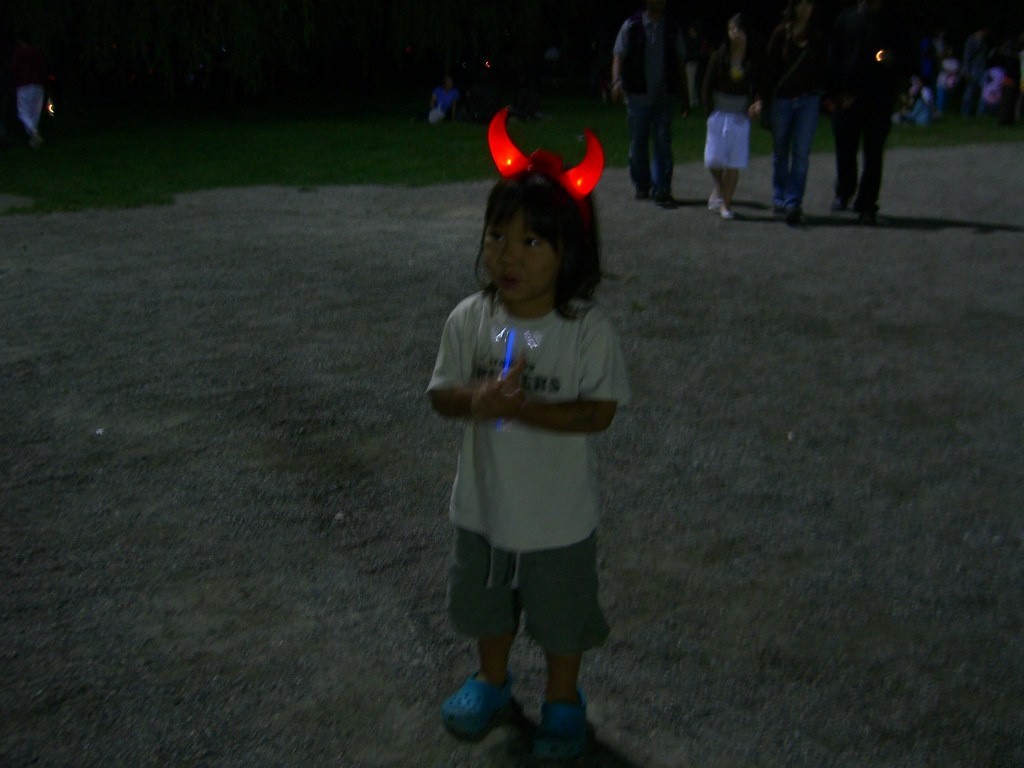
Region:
[828,189,846,209]
[856,207,880,226]
[707,195,724,214]
[652,189,673,208]
[771,206,786,218]
[634,190,650,202]
[719,207,734,219]
[783,204,802,226]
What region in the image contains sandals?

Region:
[441,666,513,735]
[532,688,587,765]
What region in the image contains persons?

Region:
[682,0,1024,129]
[428,72,546,124]
[700,12,762,220]
[823,0,901,225]
[612,0,693,211]
[426,168,630,768]
[767,0,828,226]
[11,37,54,150]
